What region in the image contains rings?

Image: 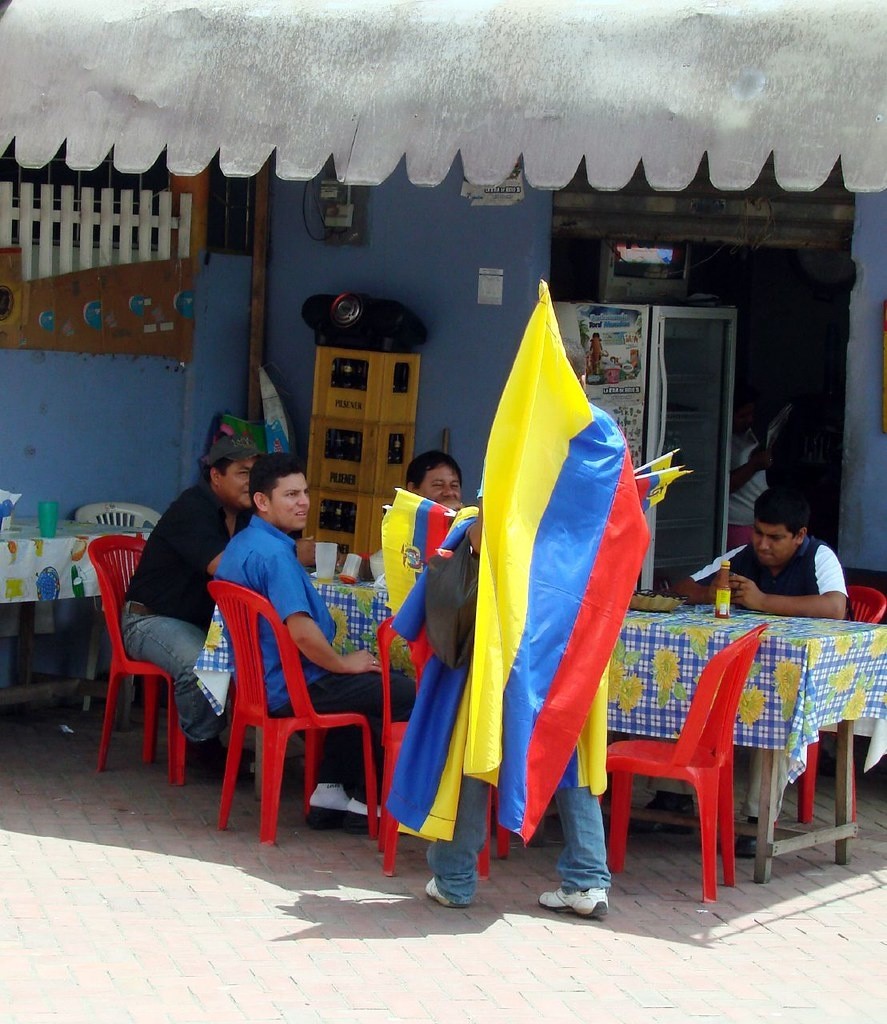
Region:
[768,456,773,461]
[373,660,376,664]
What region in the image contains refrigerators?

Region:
[542,296,739,598]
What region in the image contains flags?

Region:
[382,279,695,840]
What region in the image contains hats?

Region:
[208,434,266,463]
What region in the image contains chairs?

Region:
[798,584,887,825]
[599,621,769,905]
[207,580,379,847]
[87,534,190,788]
[374,614,512,882]
[75,502,163,711]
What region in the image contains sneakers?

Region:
[307,782,382,834]
[538,883,610,917]
[425,875,467,908]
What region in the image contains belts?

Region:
[129,603,149,614]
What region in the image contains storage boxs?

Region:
[312,344,422,425]
[302,488,394,555]
[306,415,416,498]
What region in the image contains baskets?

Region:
[629,589,688,612]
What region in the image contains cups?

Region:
[339,553,363,584]
[315,543,337,583]
[38,501,58,538]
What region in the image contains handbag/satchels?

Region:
[423,525,482,671]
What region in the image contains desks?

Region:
[191,565,887,885]
[0,515,154,733]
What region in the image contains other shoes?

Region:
[626,792,694,835]
[732,815,776,856]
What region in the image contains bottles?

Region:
[295,539,315,565]
[715,561,731,618]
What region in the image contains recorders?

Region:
[600,238,691,302]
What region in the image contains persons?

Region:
[427,336,651,920]
[629,484,849,859]
[729,384,774,546]
[120,434,340,782]
[405,450,464,509]
[212,451,416,836]
